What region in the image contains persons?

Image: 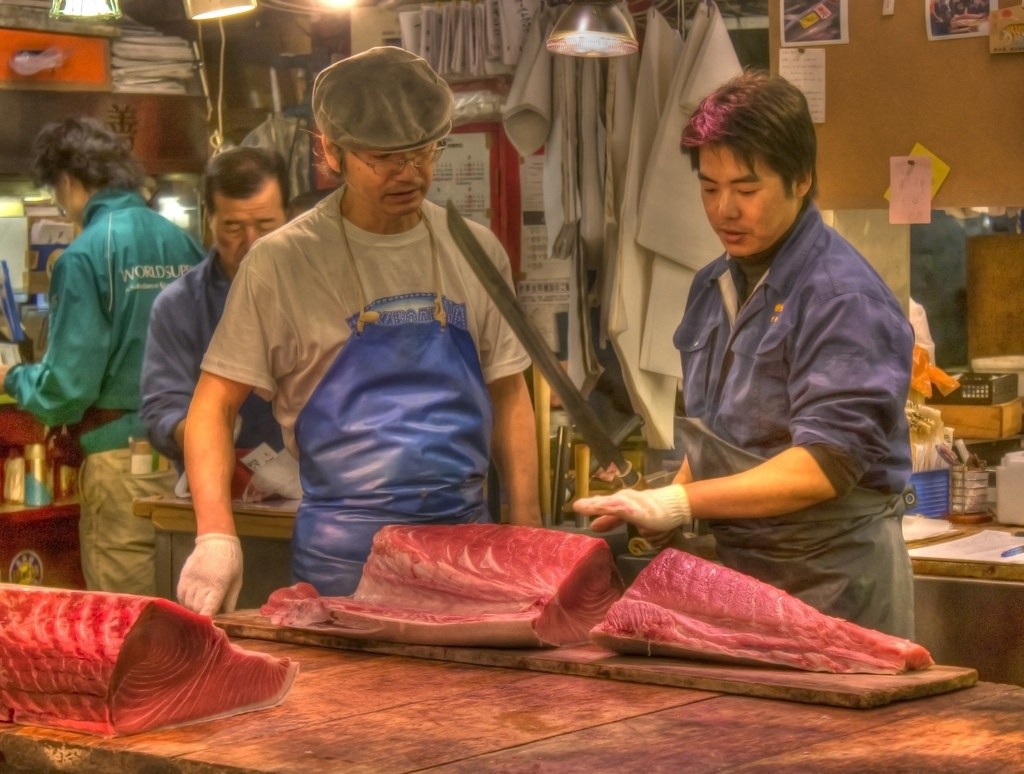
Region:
[140,146,291,490]
[175,46,546,618]
[574,74,915,646]
[0,114,207,602]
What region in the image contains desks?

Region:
[134,495,299,604]
[0,634,1024,774]
[904,515,1024,687]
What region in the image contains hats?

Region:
[311,44,456,155]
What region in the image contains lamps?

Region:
[546,0,639,58]
[48,0,123,21]
[184,0,258,19]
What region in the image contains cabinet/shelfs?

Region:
[0,404,85,591]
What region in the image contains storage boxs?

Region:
[925,373,1017,404]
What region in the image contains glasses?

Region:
[331,138,446,177]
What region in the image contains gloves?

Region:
[177,533,243,619]
[573,482,692,538]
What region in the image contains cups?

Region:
[993,449,1024,527]
[22,442,54,508]
